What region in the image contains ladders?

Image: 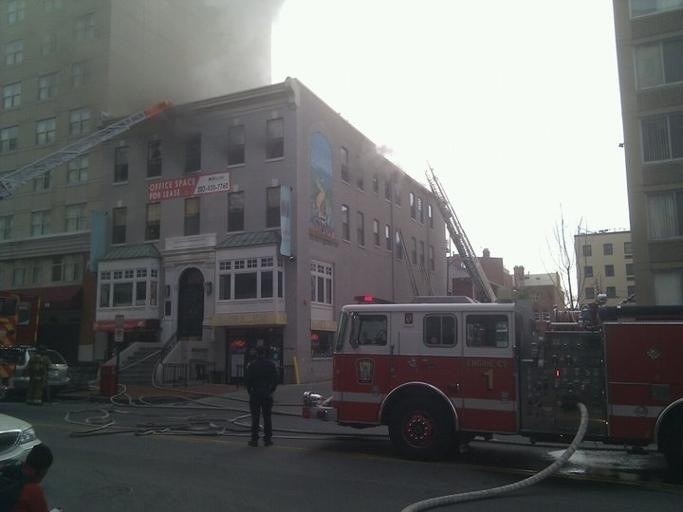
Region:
[400,231,419,297]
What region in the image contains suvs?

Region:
[1,345,72,400]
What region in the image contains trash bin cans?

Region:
[100,365,115,397]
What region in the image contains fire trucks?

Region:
[300,292,682,473]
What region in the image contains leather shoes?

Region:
[263,441,273,446]
[247,440,256,447]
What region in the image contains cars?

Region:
[0,412,54,499]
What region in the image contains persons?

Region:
[0,443,53,511]
[0,360,16,400]
[24,344,48,404]
[244,345,282,447]
